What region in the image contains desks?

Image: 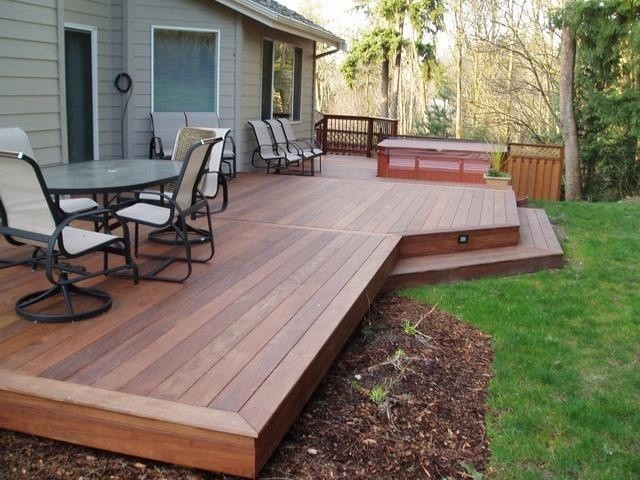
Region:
[40,159,185,284]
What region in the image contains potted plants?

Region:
[483,133,519,188]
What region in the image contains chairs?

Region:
[0,151,120,323]
[248,116,325,176]
[110,137,222,282]
[137,126,231,220]
[149,112,186,159]
[186,111,236,180]
[2,127,99,216]
